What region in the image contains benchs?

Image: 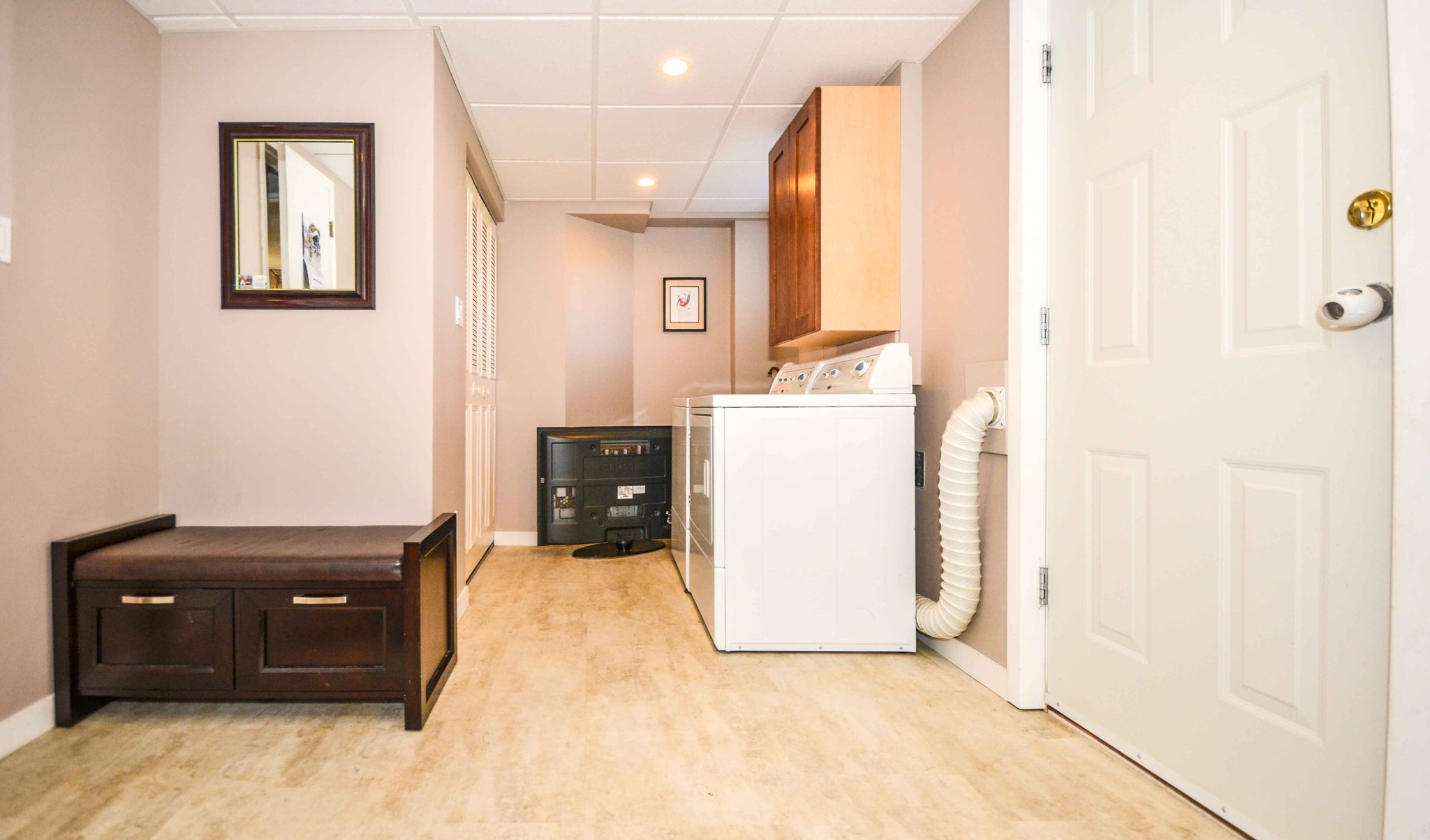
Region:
[44,500,463,731]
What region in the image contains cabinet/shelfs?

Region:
[767,84,901,355]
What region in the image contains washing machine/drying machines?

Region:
[669,338,920,656]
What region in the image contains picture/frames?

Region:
[664,275,709,333]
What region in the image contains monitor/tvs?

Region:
[534,426,674,559]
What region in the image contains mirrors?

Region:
[218,117,378,314]
